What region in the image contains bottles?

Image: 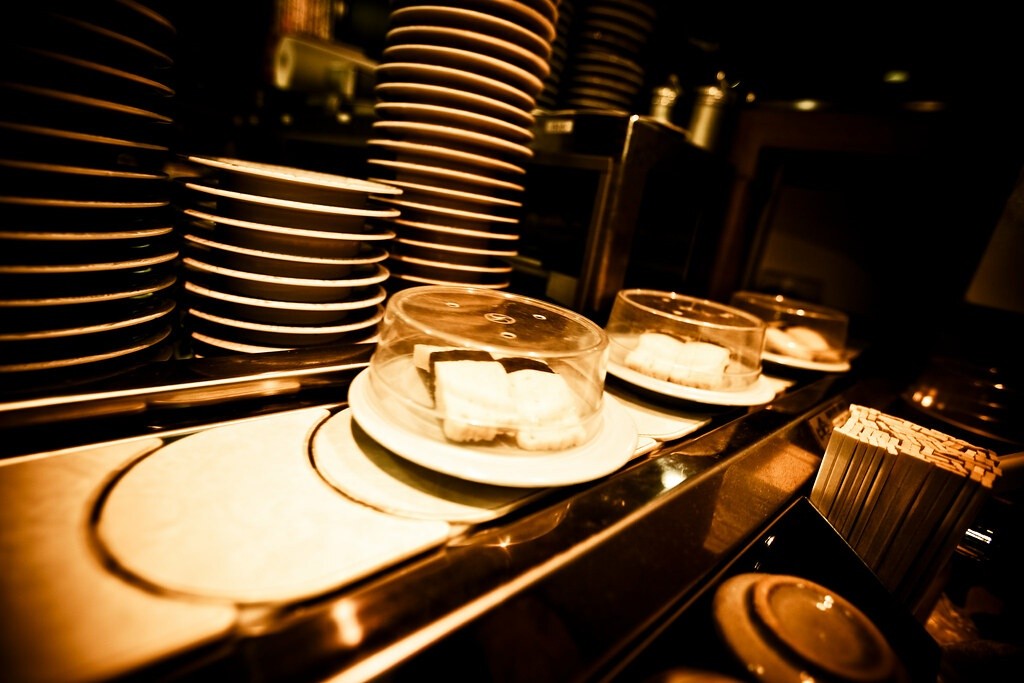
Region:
[653,88,677,123]
[691,85,726,144]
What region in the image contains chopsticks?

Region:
[809,402,1000,630]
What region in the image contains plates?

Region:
[348,356,638,490]
[708,327,852,373]
[1,0,657,393]
[606,332,775,408]
[712,572,909,683]
